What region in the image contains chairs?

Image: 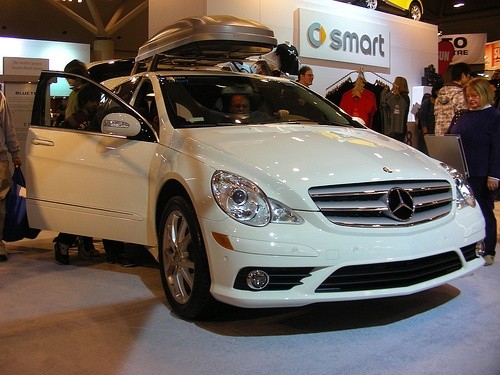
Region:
[148,101,193,129]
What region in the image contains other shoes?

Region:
[0,246,8,261]
[77,239,100,262]
[120,255,138,267]
[483,255,494,266]
[53,242,71,265]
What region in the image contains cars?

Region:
[24,15,487,320]
[338,0,425,21]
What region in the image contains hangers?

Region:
[374,74,401,96]
[326,74,352,95]
[352,75,366,98]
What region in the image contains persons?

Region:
[391,76,410,142]
[434,62,472,136]
[296,66,314,88]
[449,78,500,265]
[0,85,22,260]
[51,61,88,247]
[417,86,441,154]
[275,41,299,75]
[54,84,103,265]
[226,59,250,74]
[488,69,500,109]
[228,94,290,125]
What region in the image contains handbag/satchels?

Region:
[1,167,42,242]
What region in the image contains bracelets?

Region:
[424,132,427,135]
